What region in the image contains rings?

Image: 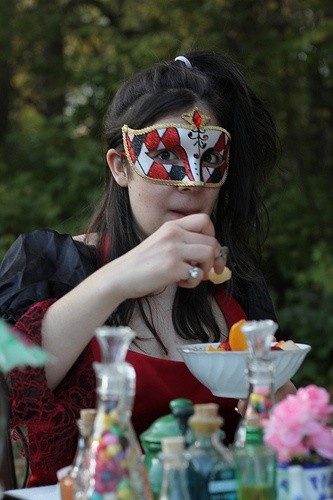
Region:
[214,247,230,260]
[188,267,199,282]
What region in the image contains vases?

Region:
[275,459,333,500]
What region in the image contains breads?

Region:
[208,266,232,284]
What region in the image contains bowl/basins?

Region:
[176,343,311,400]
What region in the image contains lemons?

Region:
[229,319,251,351]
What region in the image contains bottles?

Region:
[60,326,277,500]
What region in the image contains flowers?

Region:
[260,384,333,464]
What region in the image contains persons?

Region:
[0,50,297,489]
